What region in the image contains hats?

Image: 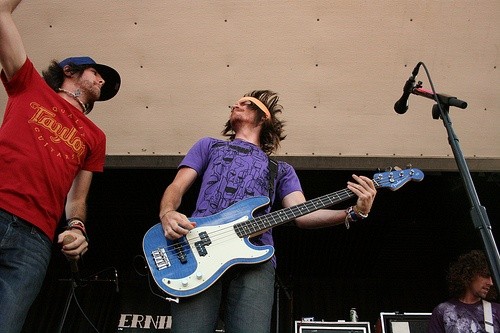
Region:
[60,57,121,102]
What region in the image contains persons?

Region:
[159,90,376,333]
[430,250,500,333]
[0,0,121,333]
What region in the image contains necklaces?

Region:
[59,88,87,114]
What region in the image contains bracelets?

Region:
[345,205,369,229]
[160,209,176,221]
[66,217,86,236]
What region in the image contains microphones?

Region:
[63,236,80,280]
[394,62,422,114]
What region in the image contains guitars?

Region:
[142,165,425,298]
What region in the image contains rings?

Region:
[78,253,83,260]
